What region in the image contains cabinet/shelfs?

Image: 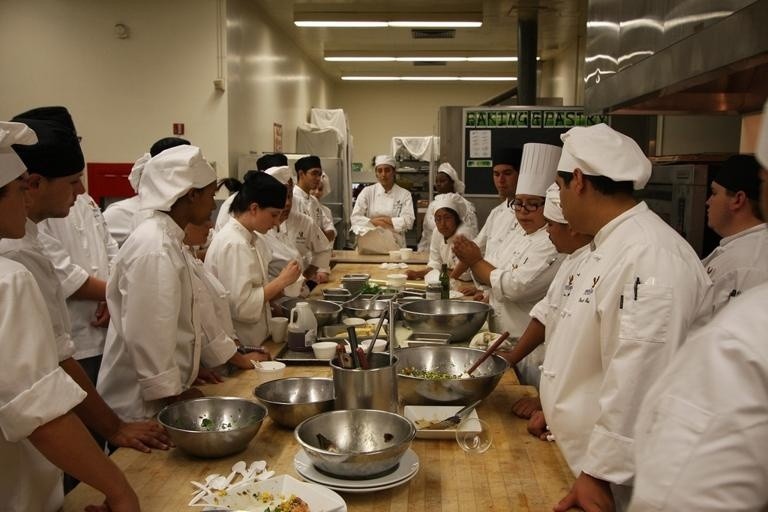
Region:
[392,136,434,211]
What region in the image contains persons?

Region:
[8,106,177,493]
[526,123,714,512]
[700,153,768,313]
[349,154,416,255]
[417,123,592,441]
[97,140,338,454]
[0,120,141,512]
[39,192,120,387]
[626,105,767,512]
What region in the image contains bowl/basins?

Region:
[386,347,510,405]
[294,408,417,478]
[277,296,493,365]
[456,418,493,454]
[384,273,409,285]
[251,376,334,428]
[156,396,268,459]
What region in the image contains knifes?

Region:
[420,399,483,430]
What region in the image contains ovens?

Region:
[638,164,709,260]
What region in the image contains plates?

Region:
[201,474,348,512]
[403,405,482,440]
[292,449,420,494]
[448,290,463,300]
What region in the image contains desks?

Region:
[329,249,428,264]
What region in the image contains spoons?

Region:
[188,461,276,507]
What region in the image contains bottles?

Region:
[439,264,450,300]
[397,148,410,161]
[426,281,442,301]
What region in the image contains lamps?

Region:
[340,72,517,81]
[322,49,517,62]
[292,11,481,28]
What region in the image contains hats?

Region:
[714,155,759,200]
[432,123,653,225]
[375,155,396,168]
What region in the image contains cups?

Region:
[271,317,289,344]
[389,250,401,261]
[330,352,400,413]
[254,362,285,384]
[400,248,413,260]
